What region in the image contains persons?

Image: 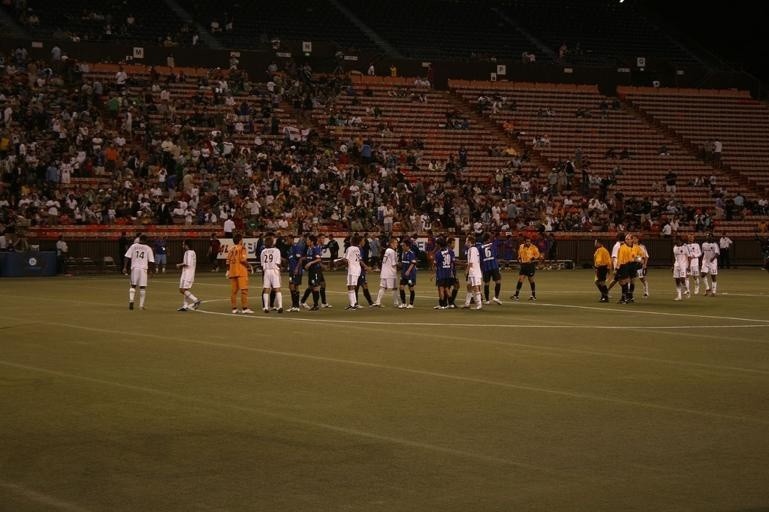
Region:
[345,233,504,311]
[672,233,720,301]
[510,236,540,301]
[226,234,255,313]
[593,233,649,304]
[122,235,154,310]
[177,238,202,312]
[260,236,333,314]
[3,3,768,273]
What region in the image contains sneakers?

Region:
[345,301,414,310]
[510,295,519,301]
[177,299,200,311]
[232,301,332,313]
[674,288,716,301]
[129,301,145,310]
[434,296,502,309]
[528,296,536,301]
[598,293,648,304]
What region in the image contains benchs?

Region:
[0,61,769,240]
